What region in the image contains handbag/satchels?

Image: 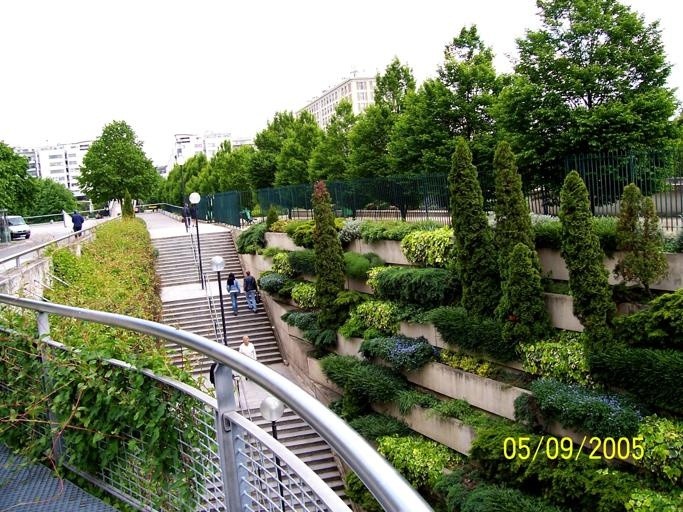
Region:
[255,296,260,303]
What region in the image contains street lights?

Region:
[177,156,185,207]
[210,256,228,346]
[188,192,205,290]
[260,395,285,512]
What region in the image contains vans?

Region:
[0,216,31,239]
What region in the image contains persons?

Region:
[182,203,191,231]
[72,210,84,238]
[243,271,259,313]
[239,335,258,381]
[227,272,240,315]
[190,204,198,227]
[95,209,109,219]
[209,360,235,387]
